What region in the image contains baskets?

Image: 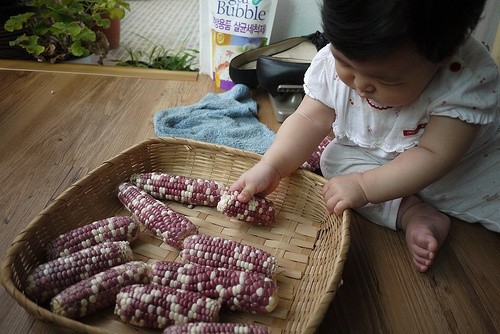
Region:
[0,136,353,334]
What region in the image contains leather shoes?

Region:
[228,30,330,97]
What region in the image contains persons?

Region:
[223,0,500,273]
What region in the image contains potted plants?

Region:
[0,0,136,64]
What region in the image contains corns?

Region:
[24,171,281,334]
